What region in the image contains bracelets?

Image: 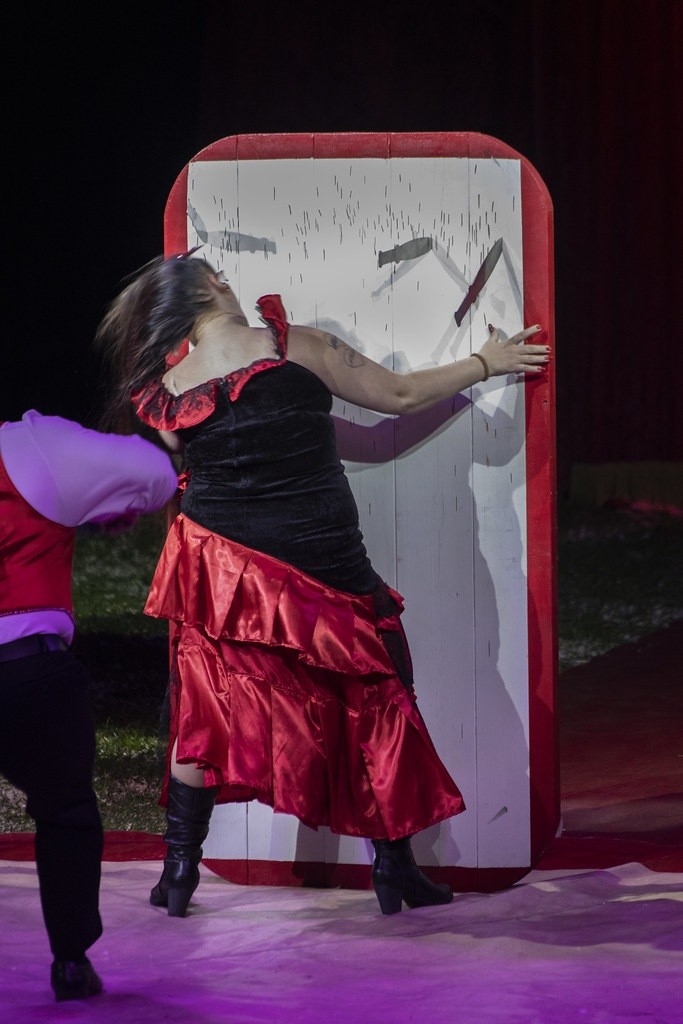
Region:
[469,353,488,381]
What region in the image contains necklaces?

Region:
[197,312,245,332]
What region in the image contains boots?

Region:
[148,775,218,917]
[371,837,454,915]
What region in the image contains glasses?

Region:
[214,269,229,285]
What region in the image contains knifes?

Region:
[185,201,207,243]
[453,238,502,328]
[378,238,432,268]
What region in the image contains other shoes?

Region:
[50,955,102,999]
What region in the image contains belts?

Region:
[1,635,66,664]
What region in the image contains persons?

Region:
[97,257,553,918]
[0,409,178,1000]
